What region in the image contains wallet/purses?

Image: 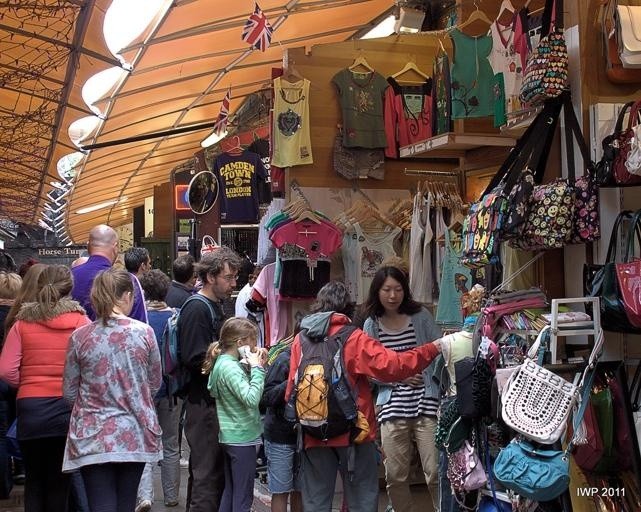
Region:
[541,311,591,324]
[522,306,570,320]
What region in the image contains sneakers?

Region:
[164,500,179,507]
[135,500,152,512]
[180,457,189,468]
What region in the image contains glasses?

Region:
[216,275,239,281]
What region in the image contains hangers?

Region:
[435,214,463,242]
[332,190,401,234]
[435,39,445,57]
[261,59,320,91]
[385,198,418,229]
[487,0,516,38]
[281,184,321,233]
[525,0,544,16]
[417,169,463,210]
[444,0,493,42]
[348,49,374,74]
[226,136,244,154]
[391,52,429,84]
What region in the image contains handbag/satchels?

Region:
[483,296,550,336]
[624,123,641,177]
[489,286,542,307]
[602,0,641,85]
[507,90,577,252]
[447,441,487,510]
[493,325,605,500]
[583,210,641,335]
[500,323,605,444]
[595,102,641,188]
[458,89,566,270]
[454,329,493,419]
[490,88,566,243]
[616,5,641,69]
[628,360,641,457]
[541,89,601,245]
[613,97,641,185]
[616,209,641,328]
[434,398,472,452]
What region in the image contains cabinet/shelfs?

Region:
[437,296,602,512]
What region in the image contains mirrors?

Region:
[188,170,219,214]
[218,223,260,298]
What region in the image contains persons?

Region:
[177,246,240,512]
[0,270,23,502]
[263,316,301,512]
[3,264,49,353]
[0,264,93,511]
[363,266,444,512]
[284,280,441,512]
[135,269,182,512]
[202,316,265,512]
[166,255,195,306]
[236,263,261,318]
[70,223,149,326]
[123,246,151,276]
[62,265,162,512]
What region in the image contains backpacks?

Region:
[163,296,215,379]
[293,321,359,438]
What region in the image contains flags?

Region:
[240,1,275,53]
[212,82,233,138]
